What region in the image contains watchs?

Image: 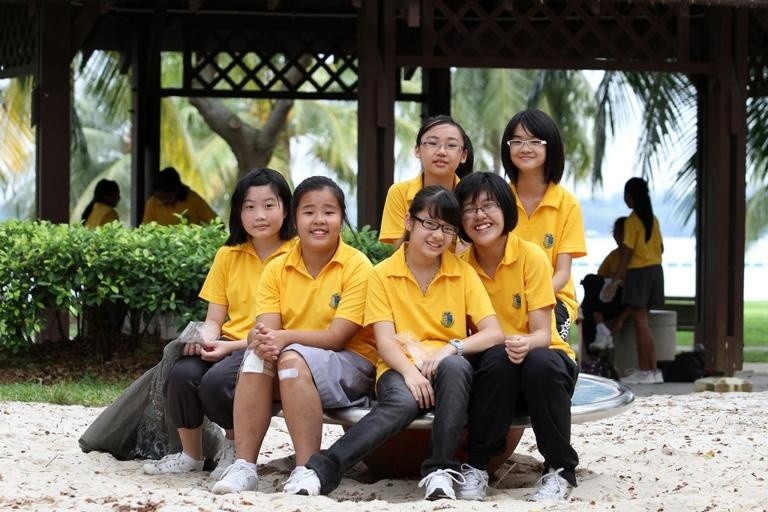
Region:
[445,338,465,361]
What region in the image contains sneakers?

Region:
[284,467,323,497]
[653,369,664,381]
[211,458,258,494]
[141,453,203,476]
[532,467,575,504]
[209,437,235,479]
[458,464,488,500]
[419,468,466,501]
[620,369,653,384]
[589,330,615,350]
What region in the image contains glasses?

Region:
[419,140,468,151]
[460,202,502,219]
[409,214,459,236]
[506,138,547,148]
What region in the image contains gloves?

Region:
[599,279,621,303]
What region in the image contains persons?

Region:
[282,185,506,502]
[454,171,580,503]
[209,176,378,494]
[81,178,122,230]
[143,166,226,232]
[600,178,666,385]
[579,217,629,381]
[380,115,474,255]
[498,110,588,343]
[143,169,297,479]
